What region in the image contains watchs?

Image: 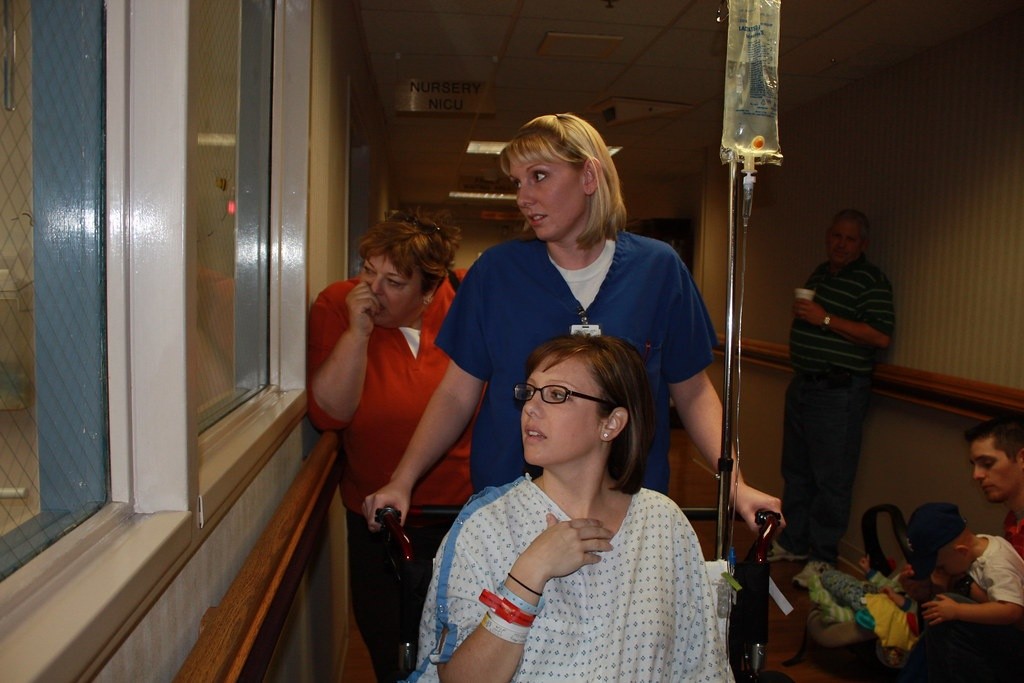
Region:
[822,313,832,330]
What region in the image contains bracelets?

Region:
[507,573,543,596]
[479,582,544,644]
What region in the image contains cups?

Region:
[795,288,816,301]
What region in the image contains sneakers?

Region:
[767,544,807,561]
[792,561,832,590]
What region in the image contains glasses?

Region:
[513,382,616,410]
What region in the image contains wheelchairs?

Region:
[369,507,795,683]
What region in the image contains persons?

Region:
[821,412,1024,683]
[304,203,489,683]
[361,113,789,537]
[409,329,739,683]
[769,204,897,589]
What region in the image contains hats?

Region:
[905,503,969,576]
